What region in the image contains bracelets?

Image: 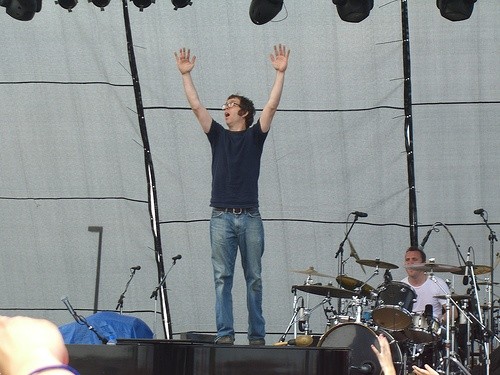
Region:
[29,364,81,375]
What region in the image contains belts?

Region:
[216,207,258,215]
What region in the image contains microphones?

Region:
[474,209,485,214]
[131,266,141,269]
[419,229,431,250]
[463,251,471,284]
[299,307,306,332]
[352,211,368,217]
[60,296,79,321]
[172,254,182,260]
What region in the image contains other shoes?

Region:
[217,335,234,345]
[248,338,266,346]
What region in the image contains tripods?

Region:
[434,300,473,374]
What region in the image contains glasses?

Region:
[221,102,241,111]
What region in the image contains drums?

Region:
[315,320,404,375]
[372,278,419,331]
[404,309,446,345]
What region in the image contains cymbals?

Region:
[403,264,463,274]
[449,264,495,275]
[432,294,474,300]
[336,275,377,302]
[284,269,335,279]
[344,232,367,275]
[475,282,500,287]
[355,259,400,270]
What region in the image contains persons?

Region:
[0,314,81,375]
[371,332,442,375]
[174,44,290,345]
[399,246,458,326]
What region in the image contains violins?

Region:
[296,285,361,299]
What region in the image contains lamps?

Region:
[436,0,477,21]
[0,0,193,21]
[332,0,374,23]
[249,0,288,25]
